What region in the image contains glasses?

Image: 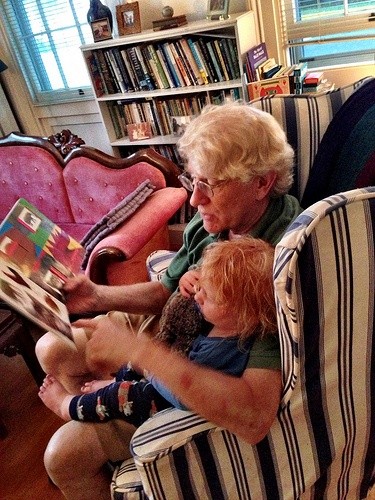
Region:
[178,169,242,198]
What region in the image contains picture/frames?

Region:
[207,0,229,19]
[116,1,141,36]
[91,17,113,43]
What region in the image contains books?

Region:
[86,33,241,98]
[118,145,184,163]
[167,192,199,226]
[105,86,240,141]
[0,196,87,352]
[242,42,336,102]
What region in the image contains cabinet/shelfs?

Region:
[79,11,257,231]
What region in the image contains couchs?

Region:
[0,130,192,285]
[111,75,375,500]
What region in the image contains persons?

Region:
[37,230,278,428]
[34,96,304,499]
